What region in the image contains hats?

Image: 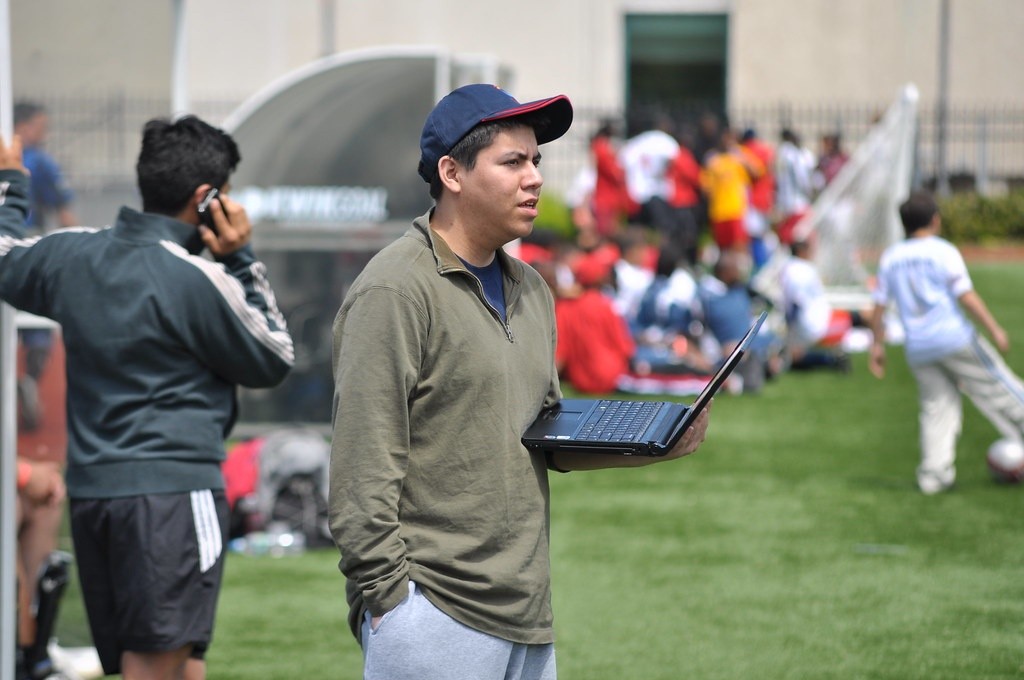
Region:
[419,84,573,184]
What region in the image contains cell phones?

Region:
[197,186,229,238]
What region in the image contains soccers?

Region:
[988,434,1023,484]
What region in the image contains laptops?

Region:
[522,310,768,456]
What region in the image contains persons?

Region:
[9,100,80,432]
[517,104,911,399]
[868,196,1024,499]
[0,116,296,680]
[18,458,65,680]
[325,84,713,679]
